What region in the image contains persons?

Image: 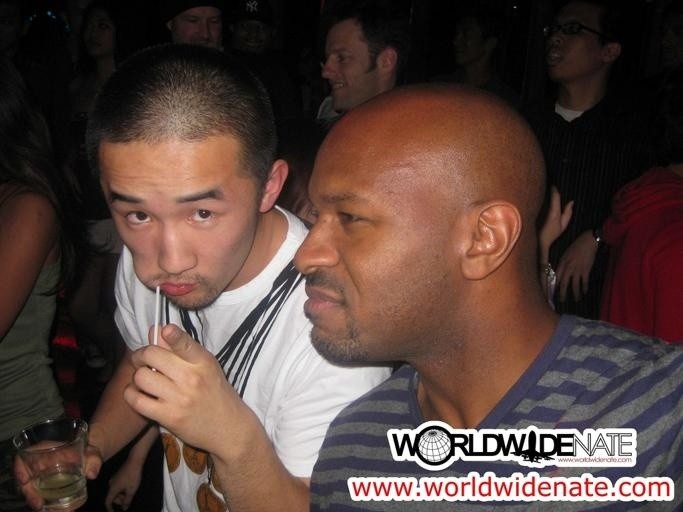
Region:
[0,0,682,511]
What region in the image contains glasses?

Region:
[542,21,601,38]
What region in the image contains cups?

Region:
[12,414,90,512]
